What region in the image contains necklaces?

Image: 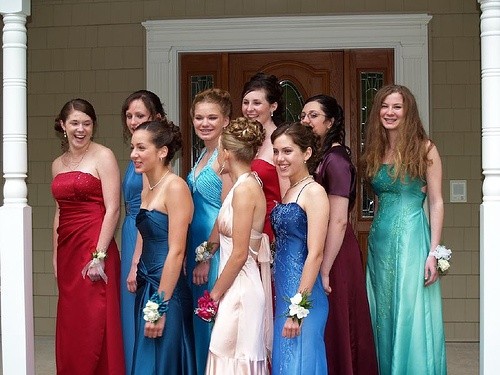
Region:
[146,168,170,191]
[255,146,262,156]
[287,174,314,187]
[66,141,92,169]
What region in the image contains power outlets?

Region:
[450,180,467,203]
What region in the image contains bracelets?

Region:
[142,289,170,323]
[81,246,108,284]
[194,290,218,323]
[281,288,313,327]
[195,240,218,262]
[428,243,452,275]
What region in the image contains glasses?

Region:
[299,112,329,122]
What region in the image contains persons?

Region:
[129,119,197,375]
[51,98,126,375]
[297,93,378,375]
[120,90,168,374]
[359,84,452,374]
[193,117,275,375]
[239,72,284,317]
[185,87,232,375]
[268,123,329,375]
